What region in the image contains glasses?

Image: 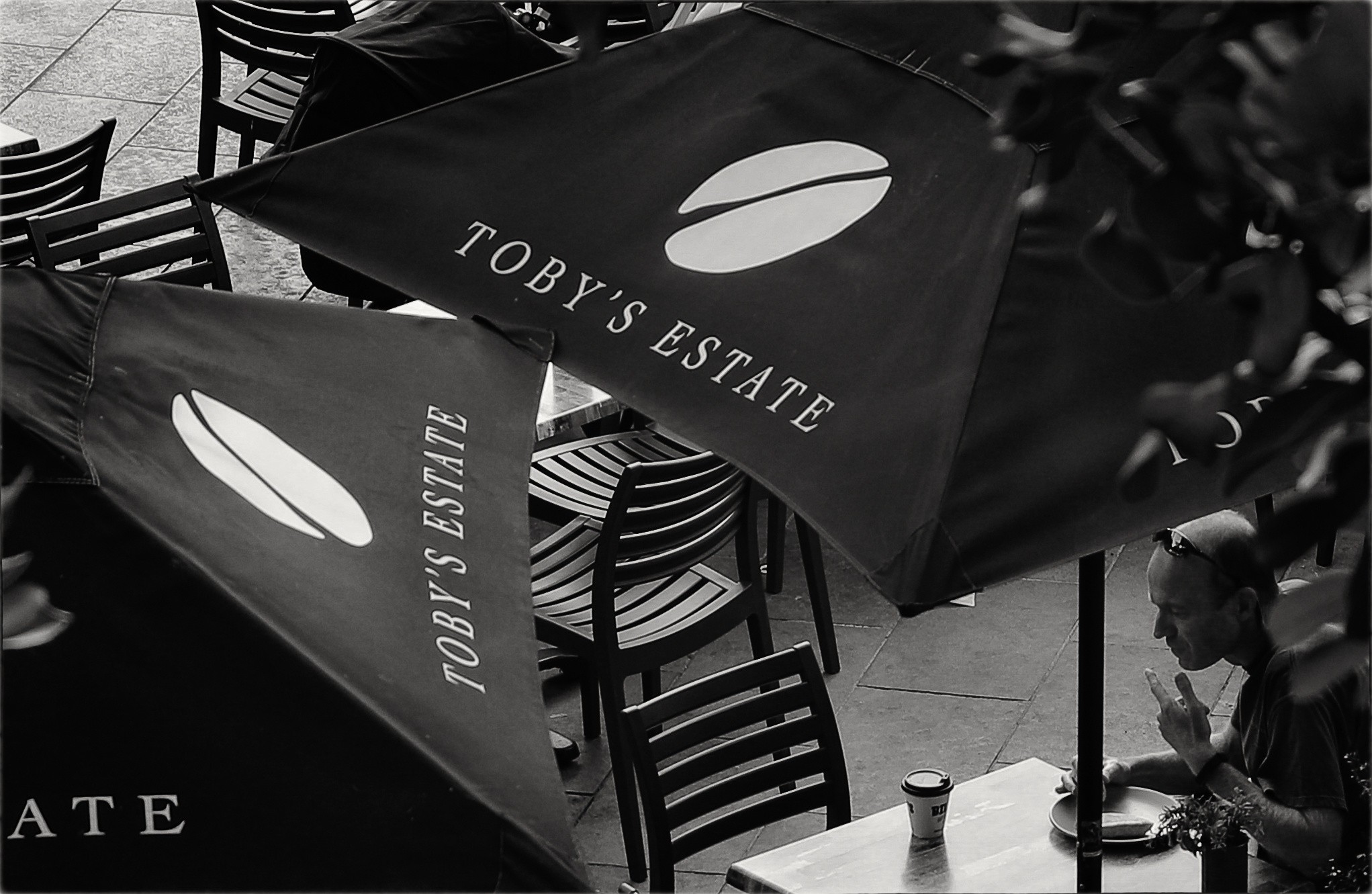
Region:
[1153,528,1245,586]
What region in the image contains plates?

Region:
[1049,786,1183,844]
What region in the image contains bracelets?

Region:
[1194,751,1228,792]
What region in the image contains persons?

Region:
[1144,511,1372,894]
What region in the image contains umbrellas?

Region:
[0,267,592,894]
[188,0,1372,894]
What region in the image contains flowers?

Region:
[1146,786,1263,860]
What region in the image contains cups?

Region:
[901,768,954,838]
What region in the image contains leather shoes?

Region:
[549,730,580,763]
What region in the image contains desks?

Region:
[729,756,1328,894]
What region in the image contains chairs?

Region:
[530,416,849,894]
[0,0,708,292]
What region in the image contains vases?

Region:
[1199,830,1249,894]
